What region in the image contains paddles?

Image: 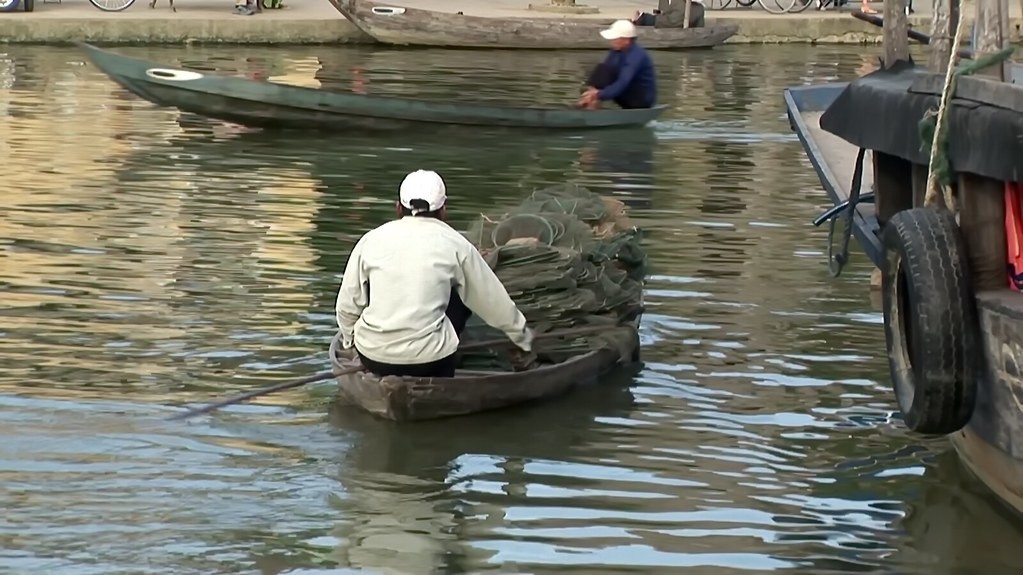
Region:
[165,336,512,420]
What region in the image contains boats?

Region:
[73,40,672,127]
[328,183,648,423]
[784,0,1023,512]
[329,0,739,49]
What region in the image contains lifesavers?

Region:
[878,204,983,440]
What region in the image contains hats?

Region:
[600,20,638,39]
[400,169,447,216]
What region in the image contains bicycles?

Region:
[699,0,846,15]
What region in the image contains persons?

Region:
[336,169,535,376]
[575,20,656,109]
